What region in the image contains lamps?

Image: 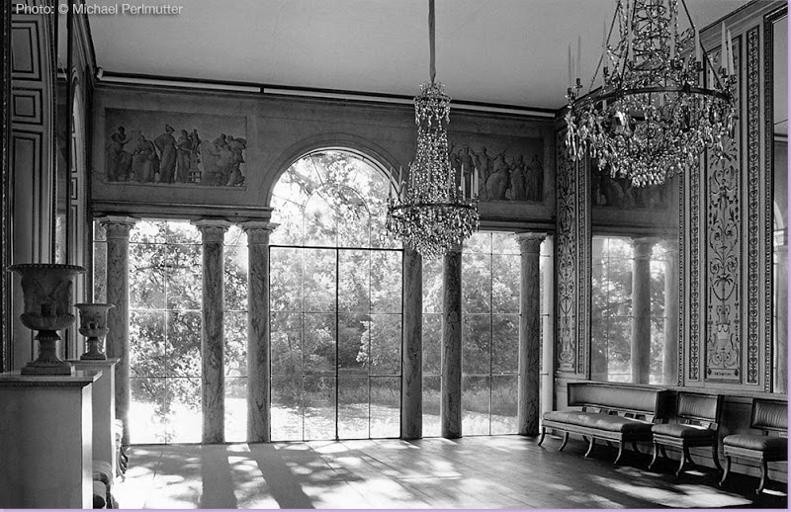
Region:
[563,1,737,188]
[386,0,480,261]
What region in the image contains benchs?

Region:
[538,383,670,464]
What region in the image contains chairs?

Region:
[648,392,787,495]
